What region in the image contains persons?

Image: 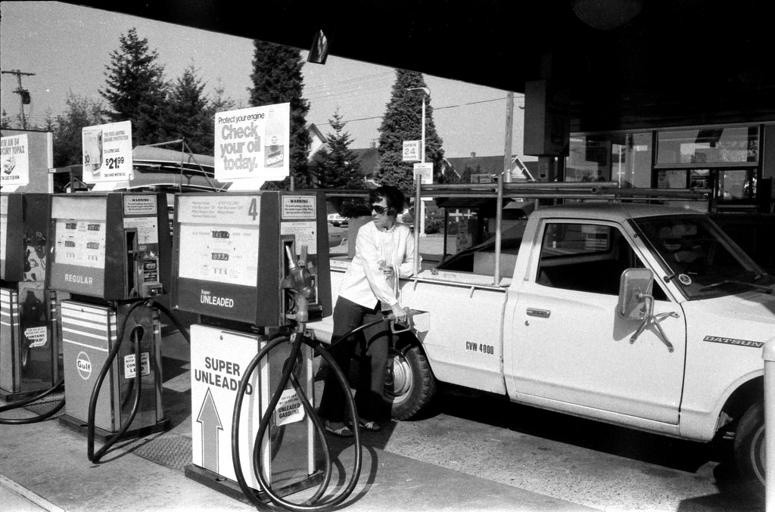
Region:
[319,186,423,437]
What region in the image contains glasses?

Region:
[368,204,388,213]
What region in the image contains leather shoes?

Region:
[325,420,380,437]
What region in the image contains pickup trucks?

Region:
[305,204,771,494]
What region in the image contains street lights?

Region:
[406,88,429,178]
